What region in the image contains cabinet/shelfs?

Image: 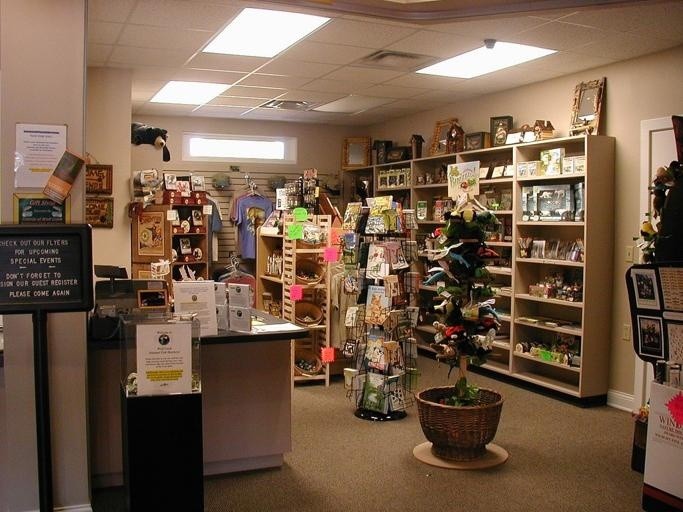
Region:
[248,210,336,388]
[129,203,214,305]
[341,132,616,403]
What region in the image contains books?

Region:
[227,305,251,331]
[227,283,250,307]
[337,194,422,414]
[215,304,227,330]
[212,282,226,306]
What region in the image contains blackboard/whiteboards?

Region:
[0,223,94,315]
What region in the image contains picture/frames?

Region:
[567,76,608,136]
[428,118,458,156]
[84,198,115,231]
[86,164,116,194]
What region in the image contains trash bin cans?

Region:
[118,314,203,512]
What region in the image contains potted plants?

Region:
[413,188,510,466]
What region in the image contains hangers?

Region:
[241,173,264,199]
